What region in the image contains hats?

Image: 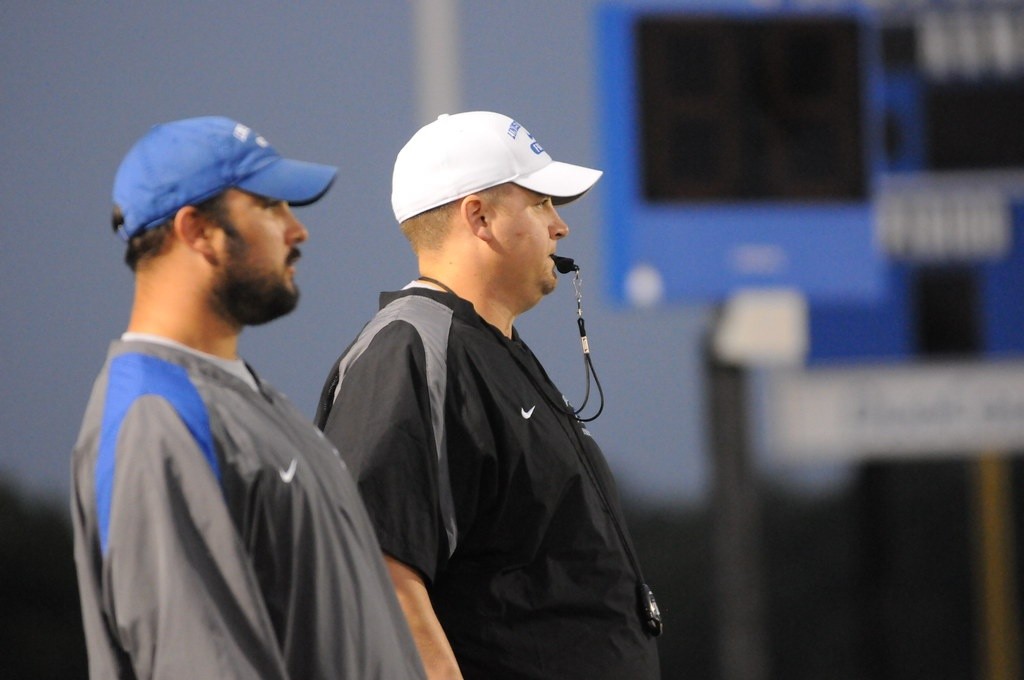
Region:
[110,115,338,244]
[391,110,604,224]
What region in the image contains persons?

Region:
[306,109,666,679]
[67,115,427,679]
